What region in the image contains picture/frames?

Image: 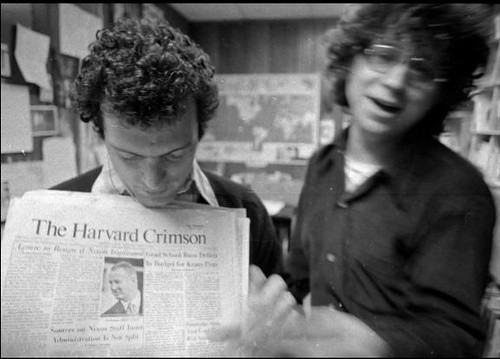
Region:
[29,104,59,137]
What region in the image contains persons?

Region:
[19,10,299,337]
[208,3,497,359]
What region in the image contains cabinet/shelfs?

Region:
[461,14,500,359]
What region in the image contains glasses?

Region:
[357,45,447,89]
